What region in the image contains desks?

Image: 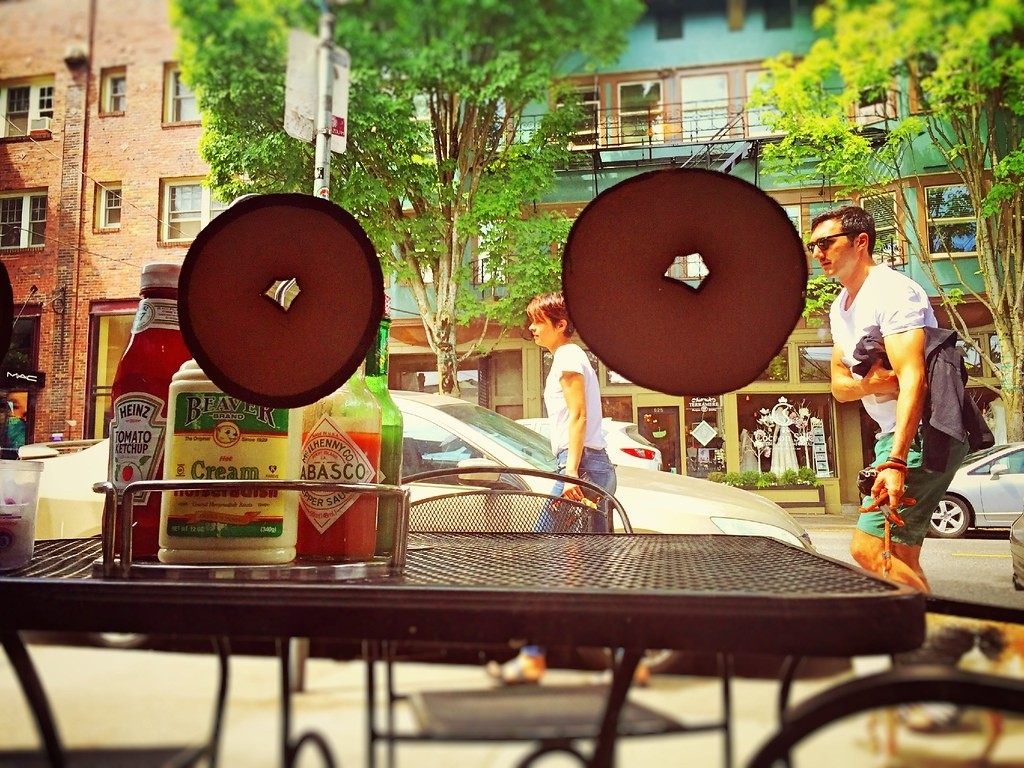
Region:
[0,531,925,768]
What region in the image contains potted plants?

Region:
[707,466,824,507]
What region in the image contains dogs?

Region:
[853,614,1024,768]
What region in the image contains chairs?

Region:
[368,466,644,768]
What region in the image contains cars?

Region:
[18,389,813,674]
[925,441,1024,539]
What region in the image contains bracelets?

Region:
[877,457,909,478]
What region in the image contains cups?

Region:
[0,459,44,571]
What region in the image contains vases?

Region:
[30,130,51,140]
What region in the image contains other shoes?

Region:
[598,668,648,685]
[487,661,540,686]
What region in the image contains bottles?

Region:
[158,350,303,565]
[295,357,381,564]
[362,317,405,557]
[100,261,191,561]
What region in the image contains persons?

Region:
[487,291,651,687]
[807,206,970,597]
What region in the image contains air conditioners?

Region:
[29,118,49,130]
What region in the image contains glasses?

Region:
[806,232,858,253]
